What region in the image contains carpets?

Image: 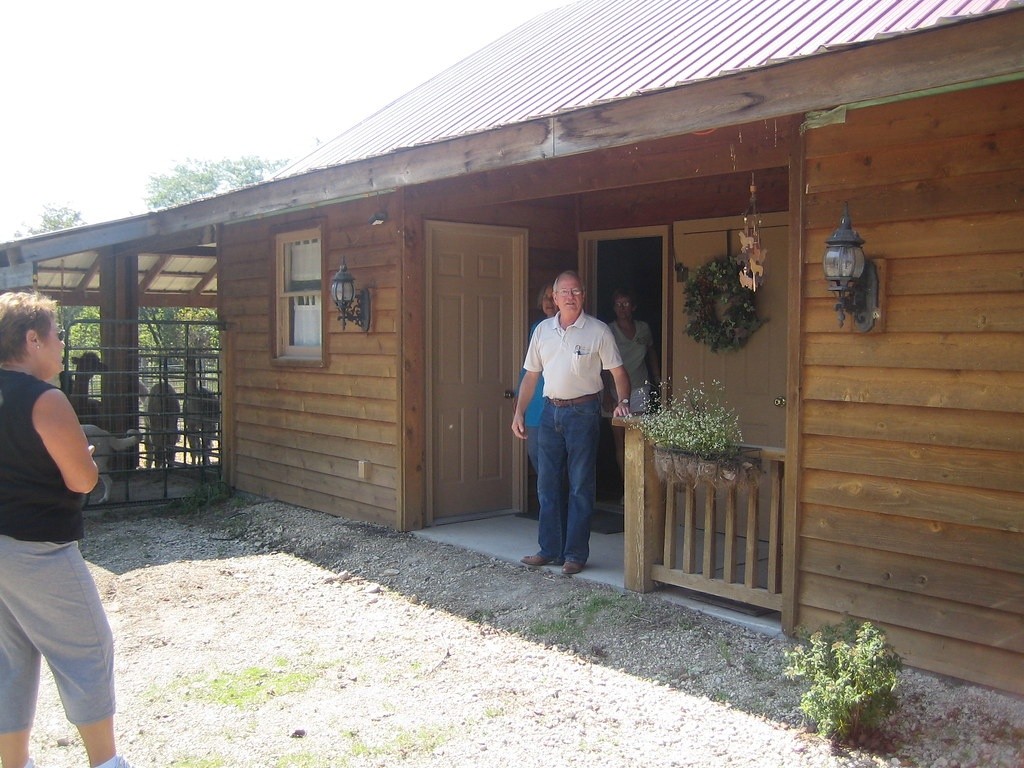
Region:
[515,504,623,533]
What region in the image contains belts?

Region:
[548,393,597,407]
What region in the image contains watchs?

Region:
[617,398,630,405]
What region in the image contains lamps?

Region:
[368,212,387,225]
[331,258,371,330]
[823,199,879,333]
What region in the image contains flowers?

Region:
[625,376,744,452]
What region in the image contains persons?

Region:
[0,289,145,768]
[512,279,559,480]
[600,286,661,507]
[512,269,633,574]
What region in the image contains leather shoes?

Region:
[520,553,563,566]
[562,560,582,574]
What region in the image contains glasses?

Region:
[555,288,583,296]
[614,301,632,306]
